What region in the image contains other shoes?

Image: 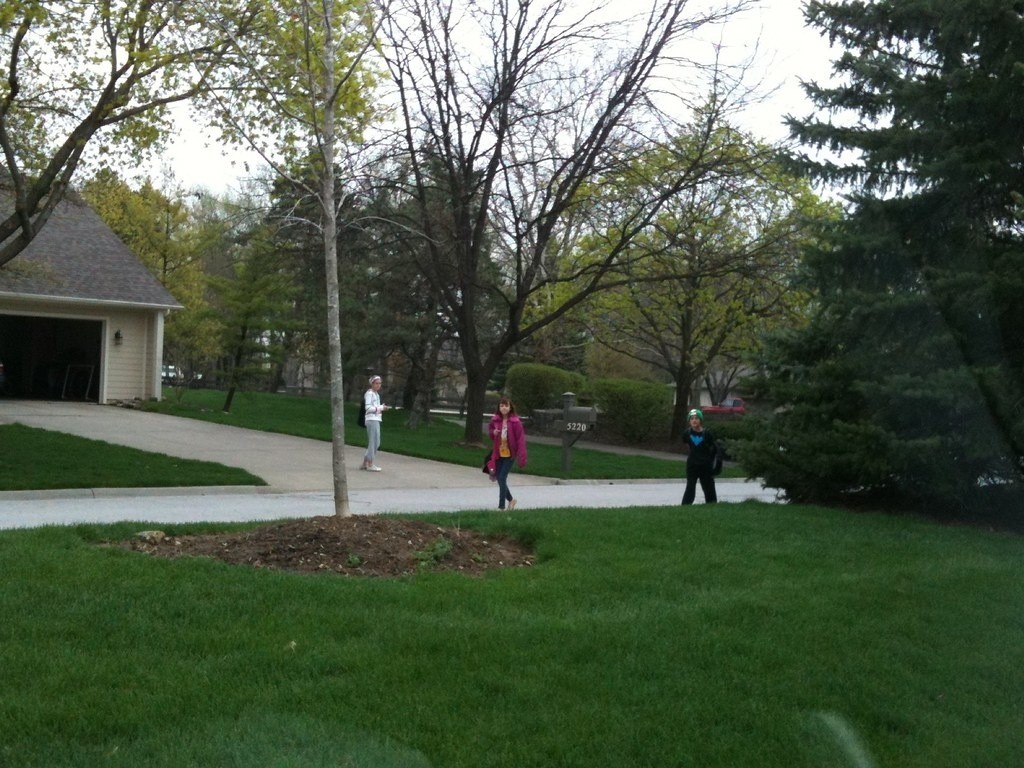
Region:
[508,500,516,510]
[360,464,369,470]
[367,464,381,472]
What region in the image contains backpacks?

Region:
[357,391,367,427]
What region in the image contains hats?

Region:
[688,409,704,422]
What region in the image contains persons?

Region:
[681,409,722,505]
[489,398,527,510]
[360,375,388,471]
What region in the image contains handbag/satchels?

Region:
[483,450,492,474]
